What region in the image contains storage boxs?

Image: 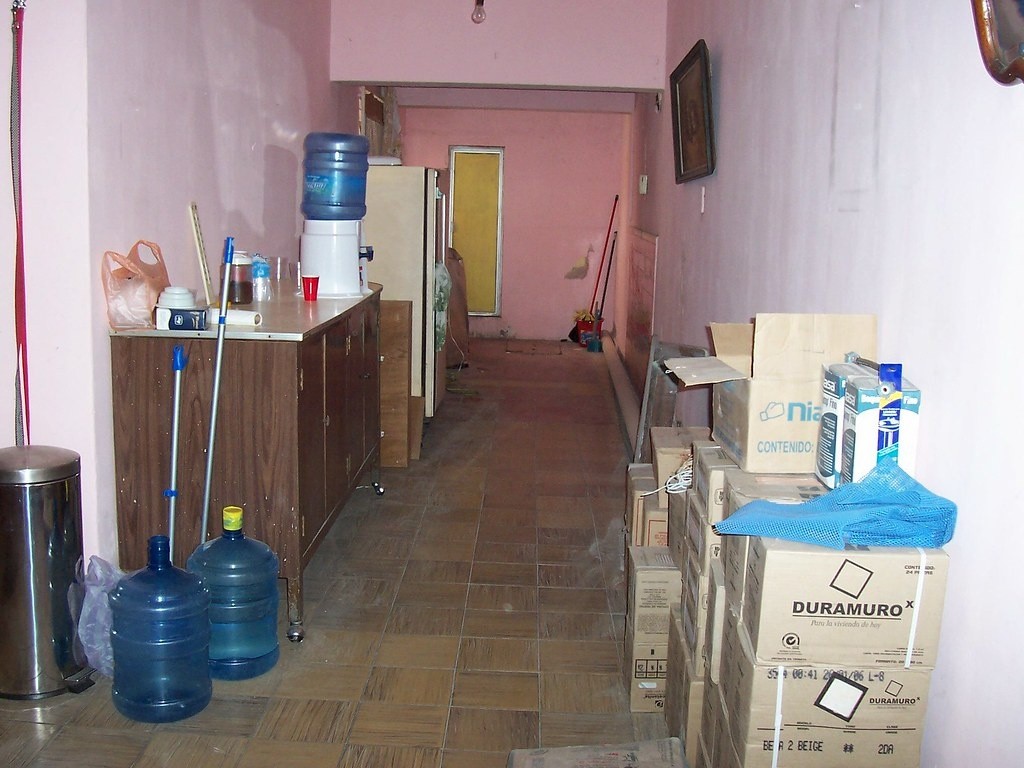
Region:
[379,299,413,468]
[501,312,950,768]
[408,394,429,462]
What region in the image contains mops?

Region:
[571,194,619,325]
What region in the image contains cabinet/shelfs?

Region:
[109,279,390,640]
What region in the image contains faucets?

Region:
[359,246,374,262]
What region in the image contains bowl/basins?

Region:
[155,286,197,310]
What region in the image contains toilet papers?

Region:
[210,308,263,327]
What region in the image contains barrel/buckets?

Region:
[575,317,605,347]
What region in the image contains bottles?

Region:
[301,131,370,221]
[220,250,253,304]
[107,535,213,724]
[187,505,280,682]
[251,253,272,303]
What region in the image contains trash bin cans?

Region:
[0,444,100,700]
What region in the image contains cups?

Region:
[301,275,320,301]
[270,256,288,280]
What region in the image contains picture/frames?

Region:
[669,36,714,185]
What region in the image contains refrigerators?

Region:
[361,167,449,418]
